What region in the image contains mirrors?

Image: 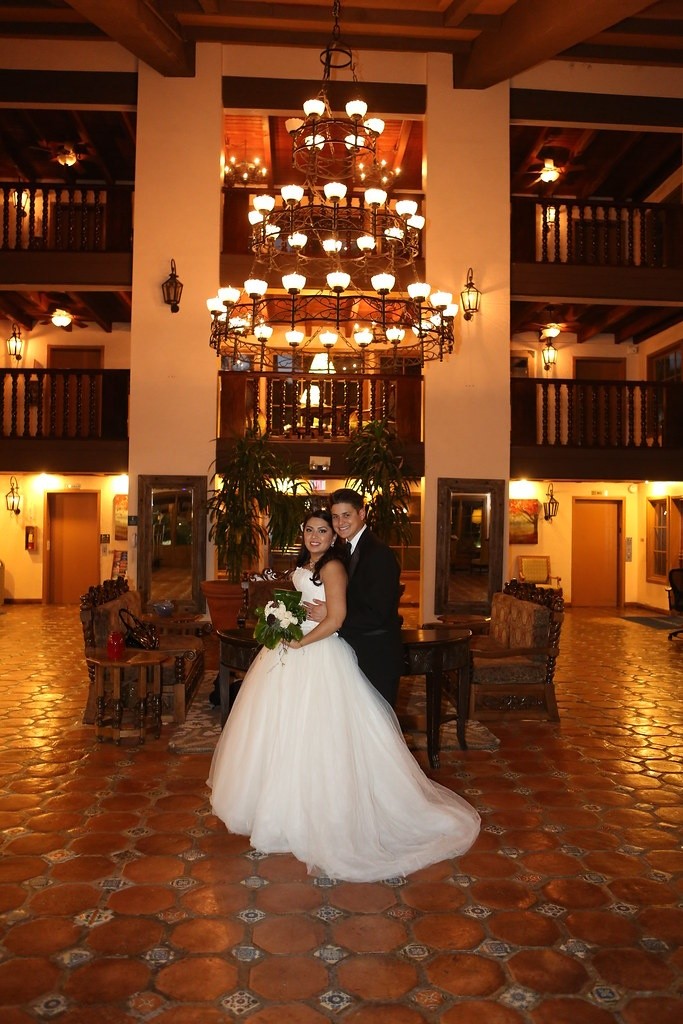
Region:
[137,474,207,615]
[434,476,504,615]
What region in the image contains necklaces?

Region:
[309,558,317,567]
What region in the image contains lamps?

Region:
[51,315,71,327]
[461,268,481,322]
[540,169,559,183]
[5,476,21,515]
[205,0,459,376]
[160,257,184,313]
[307,354,336,374]
[545,207,555,234]
[356,157,403,193]
[541,339,558,371]
[56,153,75,167]
[542,482,560,521]
[6,322,22,360]
[12,188,28,210]
[222,140,268,190]
[300,387,327,426]
[541,327,559,338]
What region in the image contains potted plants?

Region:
[345,410,421,615]
[198,423,318,634]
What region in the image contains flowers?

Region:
[254,589,309,652]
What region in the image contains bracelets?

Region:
[297,640,302,647]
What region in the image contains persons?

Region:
[299,488,401,710]
[204,510,481,884]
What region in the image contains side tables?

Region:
[84,653,165,744]
[436,613,490,634]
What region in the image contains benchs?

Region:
[441,576,566,751]
[78,575,204,727]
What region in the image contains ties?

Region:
[345,542,351,568]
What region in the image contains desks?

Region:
[217,627,472,770]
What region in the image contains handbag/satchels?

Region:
[119,609,160,650]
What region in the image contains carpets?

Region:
[625,614,683,631]
[168,668,502,758]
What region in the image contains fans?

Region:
[28,305,97,332]
[522,157,591,188]
[29,141,100,165]
[527,306,583,342]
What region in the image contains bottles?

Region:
[105,630,127,661]
[155,600,174,615]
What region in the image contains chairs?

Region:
[516,555,564,598]
[471,537,489,575]
[668,569,683,639]
